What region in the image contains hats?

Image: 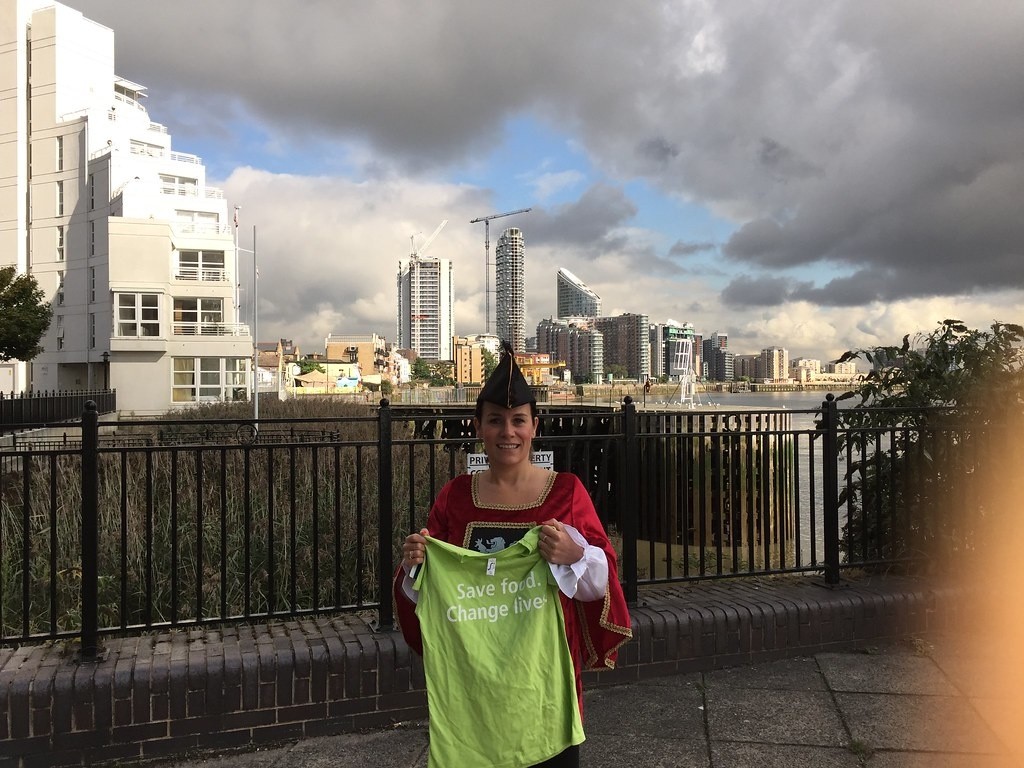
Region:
[473,355,536,408]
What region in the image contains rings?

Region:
[408,550,414,560]
[542,535,548,542]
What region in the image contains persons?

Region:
[392,379,633,768]
